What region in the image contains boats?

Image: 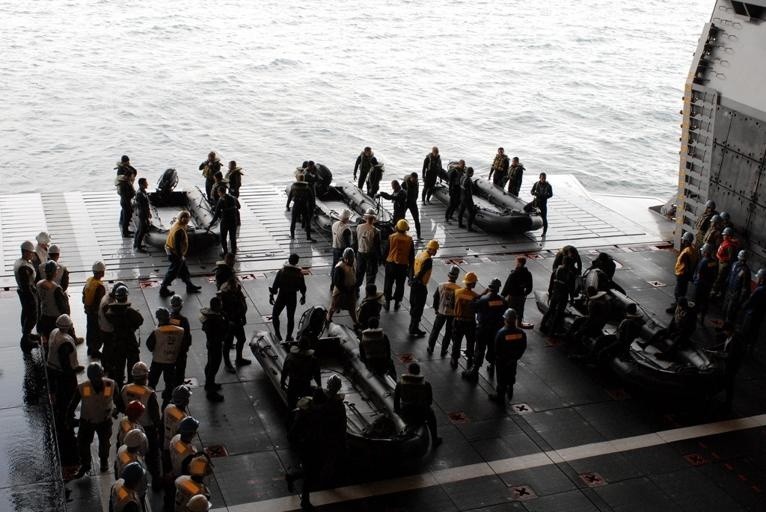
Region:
[534,287,719,383]
[249,321,432,472]
[432,175,543,235]
[131,185,222,251]
[285,178,395,243]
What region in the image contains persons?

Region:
[380,180,407,225]
[132,178,153,254]
[82,261,106,359]
[65,362,122,479]
[449,271,479,371]
[352,208,383,298]
[98,282,127,372]
[112,360,163,494]
[422,147,442,206]
[267,252,307,340]
[409,240,440,336]
[174,453,215,512]
[204,187,241,260]
[489,147,509,188]
[199,152,224,198]
[355,283,383,341]
[106,286,144,389]
[326,247,356,326]
[357,316,396,381]
[380,219,416,312]
[213,253,235,291]
[158,210,202,299]
[109,461,147,512]
[200,296,227,403]
[278,333,321,445]
[116,170,136,239]
[523,172,552,237]
[445,160,465,221]
[44,313,85,432]
[329,208,353,296]
[167,294,192,385]
[146,307,185,413]
[500,256,533,328]
[187,494,213,512]
[488,307,527,404]
[401,172,425,239]
[226,161,243,200]
[295,375,348,510]
[116,399,148,459]
[209,171,228,207]
[36,260,84,346]
[366,157,385,198]
[352,147,373,190]
[545,242,579,315]
[117,155,137,178]
[458,167,478,233]
[37,244,70,316]
[159,385,192,511]
[215,276,252,375]
[461,279,507,381]
[115,428,147,492]
[31,232,51,269]
[507,157,525,197]
[392,361,443,450]
[286,174,318,243]
[13,241,38,350]
[296,161,345,202]
[635,201,765,373]
[168,416,199,476]
[426,263,462,359]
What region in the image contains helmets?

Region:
[171,384,192,399]
[339,209,350,221]
[488,278,501,289]
[185,493,212,511]
[737,249,748,260]
[125,400,146,417]
[91,260,105,272]
[131,362,150,375]
[176,210,191,222]
[699,242,711,252]
[463,271,478,283]
[119,460,145,480]
[681,231,694,241]
[342,246,354,257]
[114,284,129,295]
[709,214,721,222]
[55,313,72,329]
[722,227,733,235]
[426,240,439,249]
[188,454,208,475]
[720,211,729,220]
[113,281,128,290]
[48,245,61,254]
[20,240,37,252]
[502,307,518,318]
[45,260,58,272]
[180,416,200,433]
[448,265,460,277]
[705,200,715,209]
[86,361,103,377]
[170,294,183,305]
[755,268,766,279]
[123,429,146,447]
[396,219,408,232]
[36,231,51,244]
[364,208,376,216]
[155,306,170,318]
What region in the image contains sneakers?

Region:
[288,233,294,239]
[461,369,477,382]
[409,326,426,336]
[285,473,295,493]
[203,382,222,391]
[158,287,175,296]
[426,346,434,353]
[450,359,458,369]
[487,365,495,372]
[487,393,506,402]
[383,302,390,310]
[186,284,202,291]
[432,436,442,448]
[206,391,224,401]
[224,363,236,374]
[394,303,401,310]
[235,358,252,364]
[440,350,447,357]
[300,501,317,511]
[307,236,317,242]
